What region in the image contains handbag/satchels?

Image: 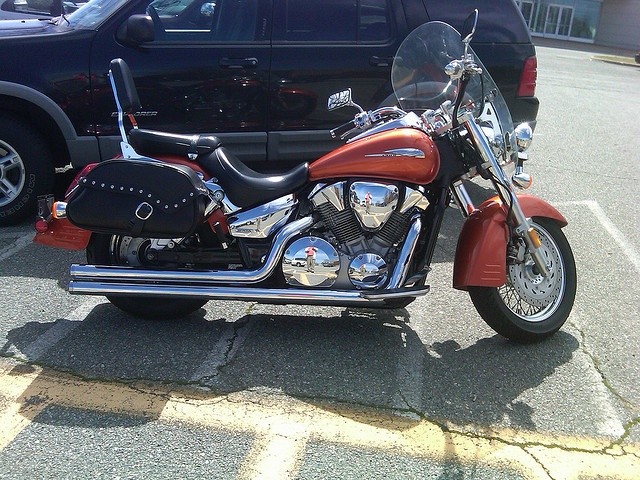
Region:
[64,156,217,241]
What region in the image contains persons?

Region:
[360,263,367,272]
[304,247,317,273]
[364,192,372,214]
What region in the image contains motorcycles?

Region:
[34,10,576,343]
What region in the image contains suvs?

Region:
[0,0,539,226]
